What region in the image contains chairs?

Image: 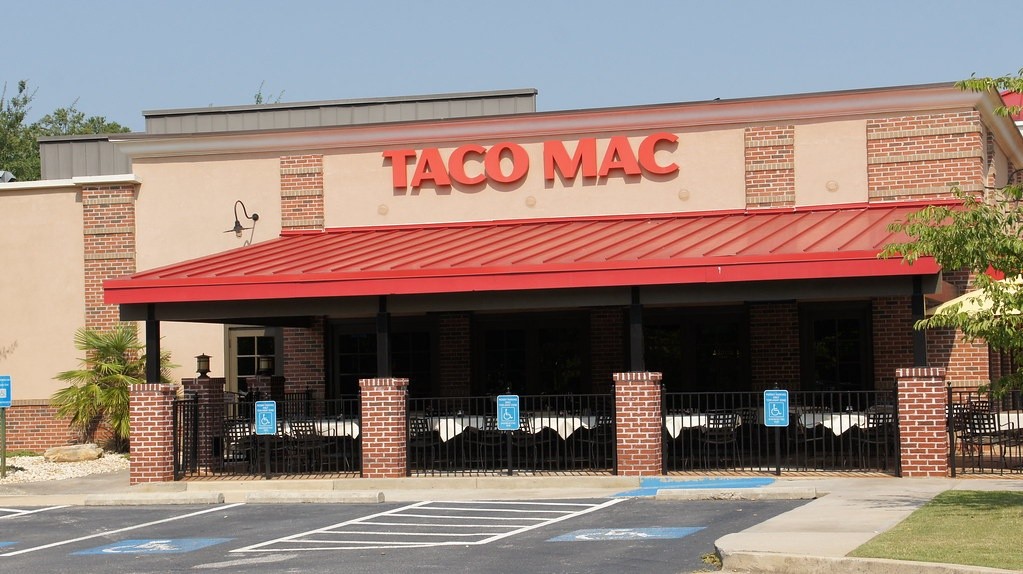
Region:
[219,394,1022,472]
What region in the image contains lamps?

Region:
[194,353,212,379]
[223,200,259,238]
[259,356,274,375]
